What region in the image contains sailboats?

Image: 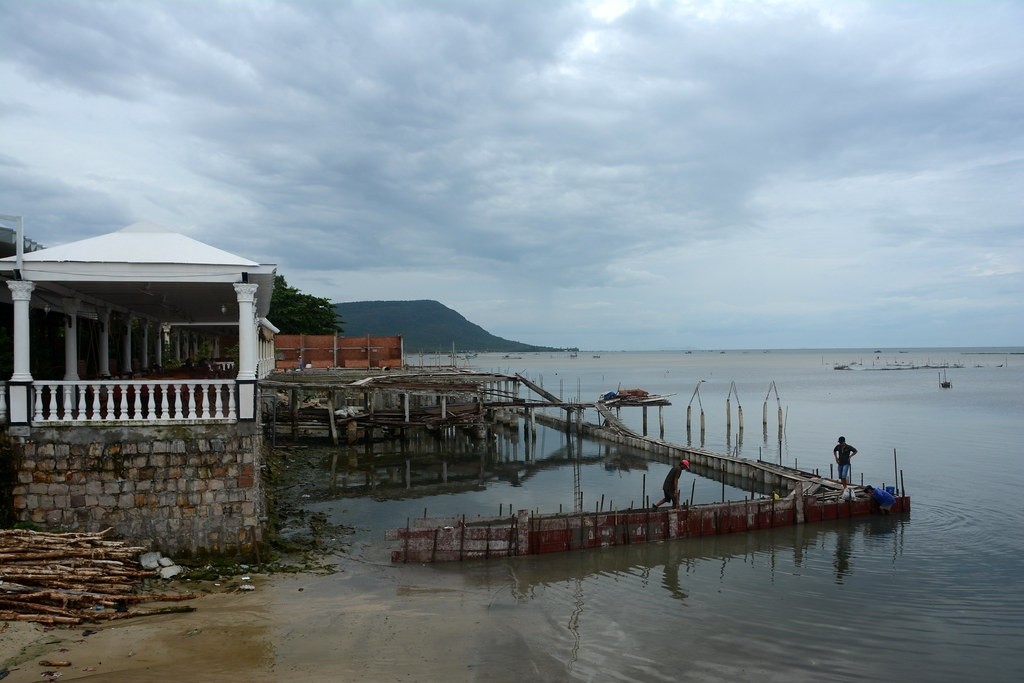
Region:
[940,364,950,388]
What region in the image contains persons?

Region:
[833,436,858,489]
[179,358,193,370]
[652,459,689,512]
[864,485,897,514]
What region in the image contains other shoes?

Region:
[652,504,657,512]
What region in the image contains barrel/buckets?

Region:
[886,486,894,495]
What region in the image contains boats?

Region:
[600,387,672,407]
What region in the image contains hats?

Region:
[681,460,689,469]
[838,437,845,442]
[864,485,873,493]
